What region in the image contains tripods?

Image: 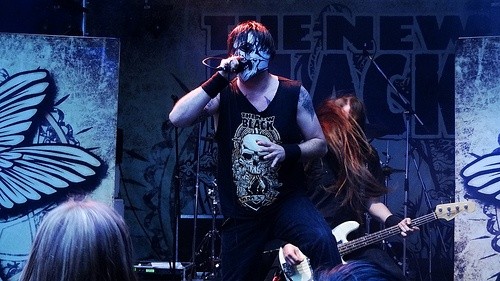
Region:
[189,185,223,277]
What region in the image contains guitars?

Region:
[278,195,477,281]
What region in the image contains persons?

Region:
[170,22,342,281]
[264,74,421,281]
[19,195,134,281]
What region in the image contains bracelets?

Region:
[385,214,401,229]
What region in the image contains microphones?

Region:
[218,62,246,73]
